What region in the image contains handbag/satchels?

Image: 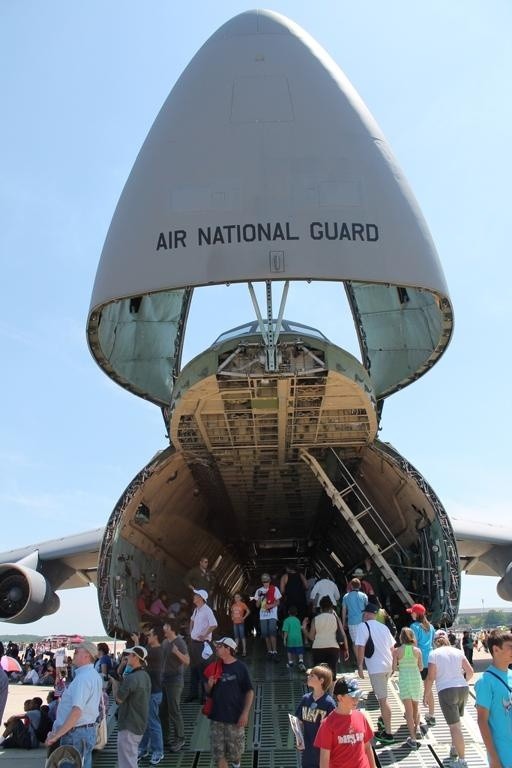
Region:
[360,621,375,658]
[93,691,107,750]
[334,614,345,641]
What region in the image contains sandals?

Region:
[242,650,247,656]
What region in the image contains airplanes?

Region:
[0,6,512,768]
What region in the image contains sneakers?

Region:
[454,759,469,768]
[299,661,307,672]
[137,736,186,764]
[266,649,278,656]
[286,662,295,669]
[373,711,436,750]
[449,746,460,756]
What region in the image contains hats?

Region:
[405,603,426,616]
[260,573,271,582]
[360,604,378,614]
[71,641,98,660]
[351,568,366,577]
[215,637,237,650]
[334,676,364,698]
[123,646,148,661]
[193,589,209,603]
[433,629,450,640]
